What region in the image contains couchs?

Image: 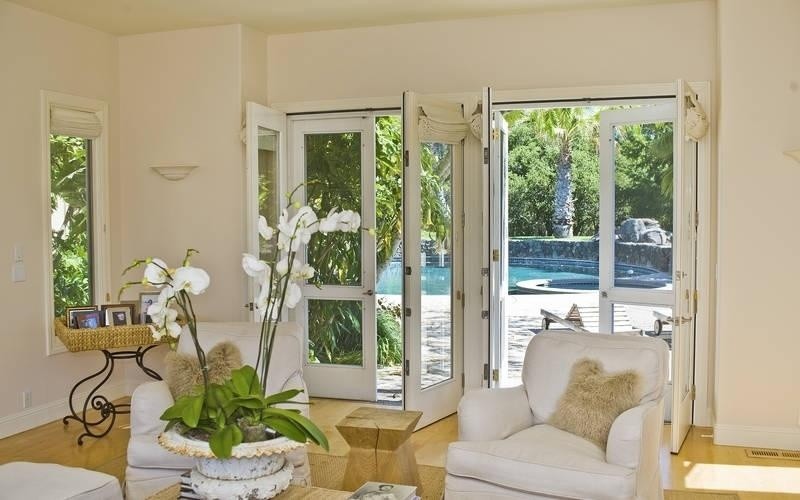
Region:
[1,460,123,499]
[124,320,311,500]
[445,329,669,499]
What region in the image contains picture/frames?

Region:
[101,299,140,328]
[139,291,162,326]
[65,304,99,329]
[75,311,102,330]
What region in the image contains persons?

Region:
[142,299,153,324]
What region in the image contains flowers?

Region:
[118,179,362,461]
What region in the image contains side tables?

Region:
[336,406,425,498]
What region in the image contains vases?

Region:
[156,421,312,499]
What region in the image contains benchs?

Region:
[539,302,640,334]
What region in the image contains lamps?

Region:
[150,165,200,180]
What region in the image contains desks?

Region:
[148,472,355,499]
[54,315,187,445]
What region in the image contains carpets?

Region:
[307,454,739,500]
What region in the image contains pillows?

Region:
[552,360,640,449]
